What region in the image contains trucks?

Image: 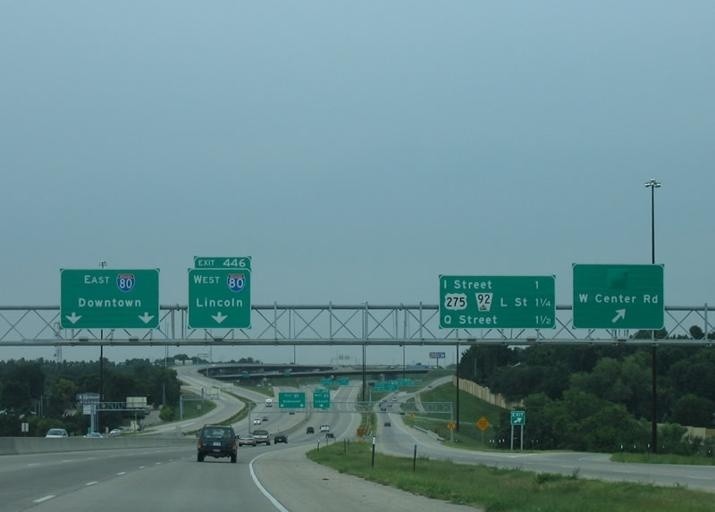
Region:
[265,398,272,407]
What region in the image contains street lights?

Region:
[643,179,662,453]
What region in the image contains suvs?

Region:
[198,423,241,463]
[253,430,270,445]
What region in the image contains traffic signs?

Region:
[186,268,252,330]
[375,379,412,392]
[430,352,445,358]
[439,273,556,330]
[509,410,525,426]
[313,390,330,408]
[194,257,252,269]
[227,273,245,292]
[320,377,350,386]
[279,393,306,409]
[59,267,159,330]
[116,273,134,292]
[570,263,664,330]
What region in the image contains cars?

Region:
[378,397,405,428]
[320,424,330,432]
[253,418,261,425]
[274,434,288,444]
[239,434,256,447]
[306,427,314,434]
[45,428,68,439]
